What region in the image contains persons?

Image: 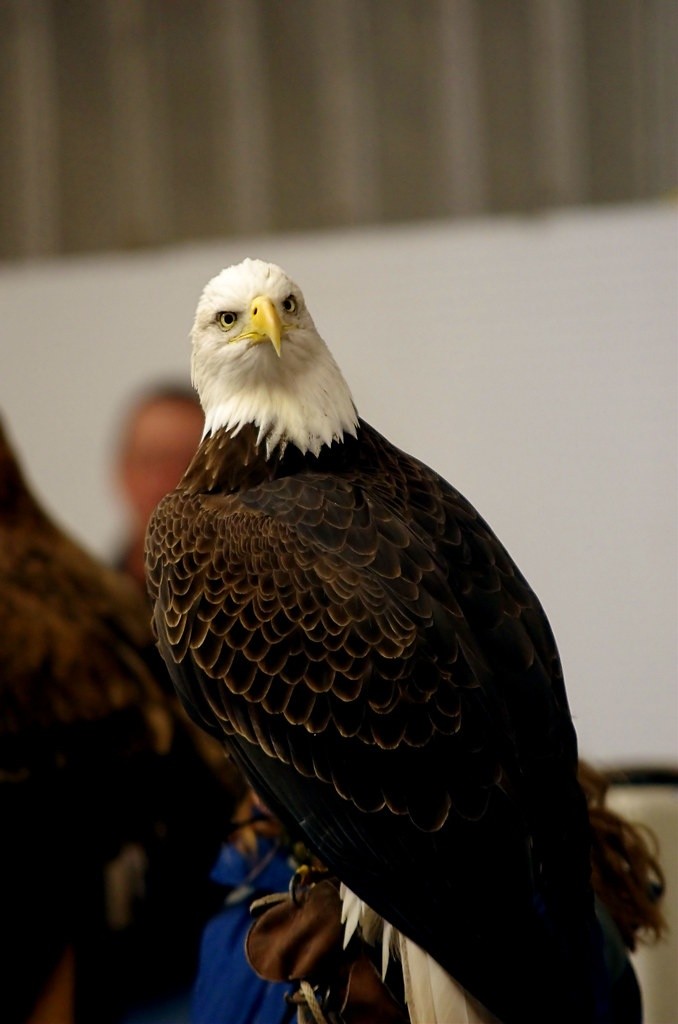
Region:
[116,378,404,1024]
